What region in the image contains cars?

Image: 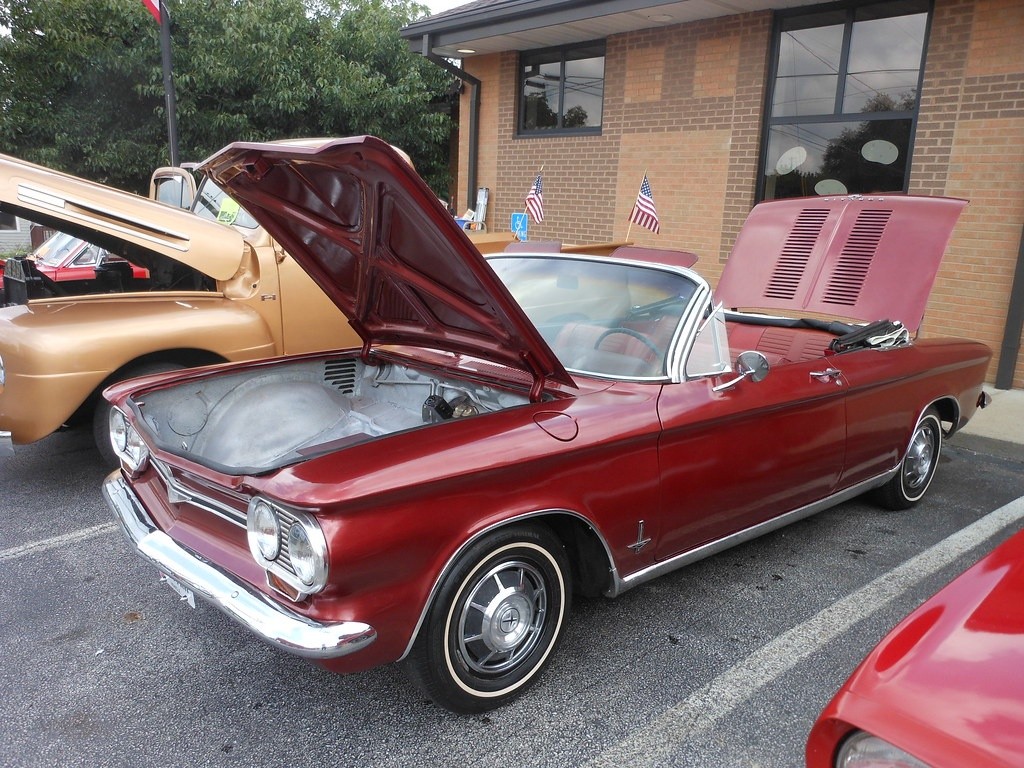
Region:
[102,131,997,712]
[805,527,1023,768]
[0,153,635,469]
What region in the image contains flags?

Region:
[142,0,161,26]
[628,176,662,234]
[525,175,544,223]
